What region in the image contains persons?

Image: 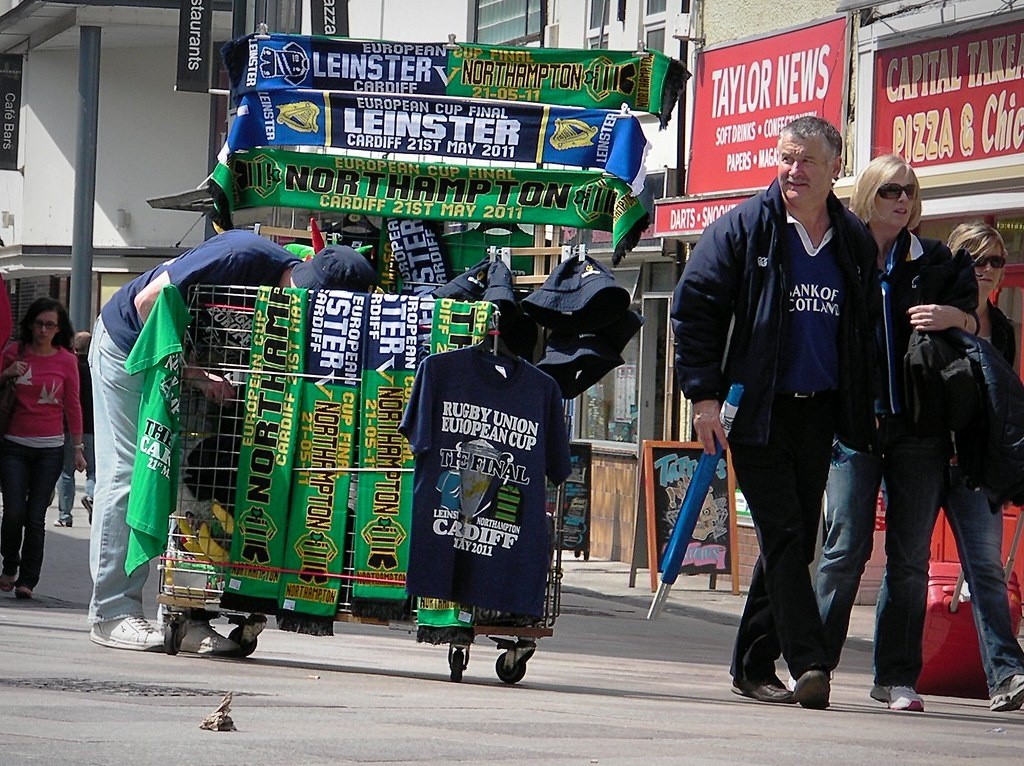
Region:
[670,116,881,712]
[813,156,980,713]
[948,219,1024,709]
[87,228,377,658]
[0,239,92,599]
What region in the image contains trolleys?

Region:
[154,282,567,685]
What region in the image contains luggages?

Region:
[916,507,1024,691]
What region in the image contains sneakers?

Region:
[870,684,924,712]
[988,674,1024,712]
[89,615,164,650]
[157,619,240,656]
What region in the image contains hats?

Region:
[431,254,645,400]
[292,244,376,294]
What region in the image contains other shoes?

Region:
[0,574,16,591]
[81,495,93,515]
[15,586,32,599]
[54,519,73,527]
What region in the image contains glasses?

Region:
[877,183,918,200]
[970,254,1006,268]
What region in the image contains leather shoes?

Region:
[730,679,798,705]
[793,670,831,710]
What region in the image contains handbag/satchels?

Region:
[0,338,24,438]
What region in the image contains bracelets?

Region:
[960,311,968,332]
